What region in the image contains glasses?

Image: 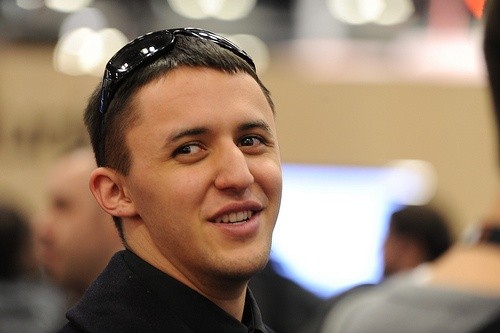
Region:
[98,27,256,138]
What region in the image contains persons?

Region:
[300,1,500,333]
[55,27,330,333]
[0,137,454,333]
